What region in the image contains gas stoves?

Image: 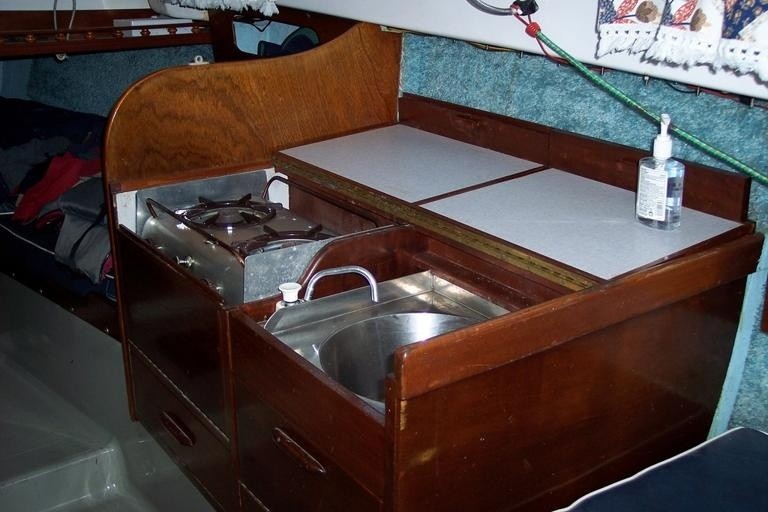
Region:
[137,175,341,309]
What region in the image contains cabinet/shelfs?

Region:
[102,22,766,512]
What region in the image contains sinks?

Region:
[264,270,511,415]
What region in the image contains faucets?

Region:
[303,266,380,306]
